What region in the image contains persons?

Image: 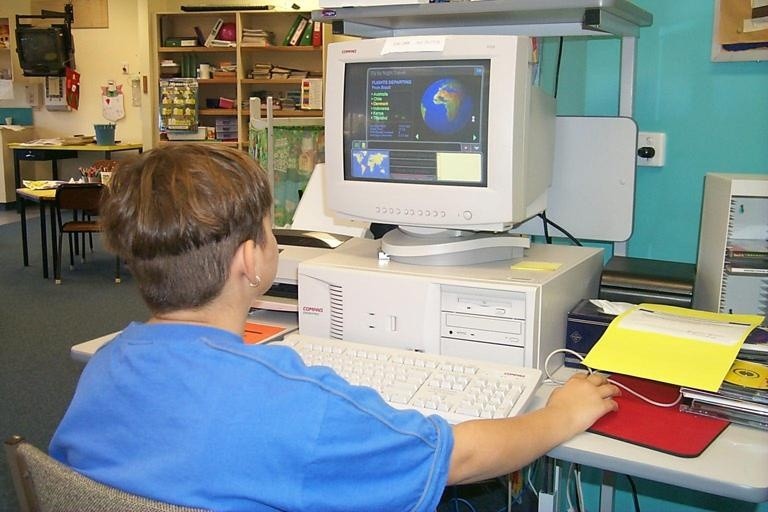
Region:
[46,142,621,512]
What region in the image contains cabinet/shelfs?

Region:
[147,1,333,153]
[0,125,34,211]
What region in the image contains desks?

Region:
[16,181,95,281]
[72,2,767,512]
[7,142,143,213]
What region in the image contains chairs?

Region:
[2,434,208,512]
[54,184,122,285]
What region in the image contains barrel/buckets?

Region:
[94,125,116,145]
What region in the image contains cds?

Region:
[724,360,768,389]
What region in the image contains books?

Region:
[239,14,324,47]
[241,88,300,110]
[246,61,322,83]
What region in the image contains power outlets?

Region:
[636,132,665,166]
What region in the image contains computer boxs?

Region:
[296,243,605,380]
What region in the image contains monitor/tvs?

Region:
[323,37,557,256]
[15,24,70,71]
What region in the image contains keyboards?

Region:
[283,338,539,429]
